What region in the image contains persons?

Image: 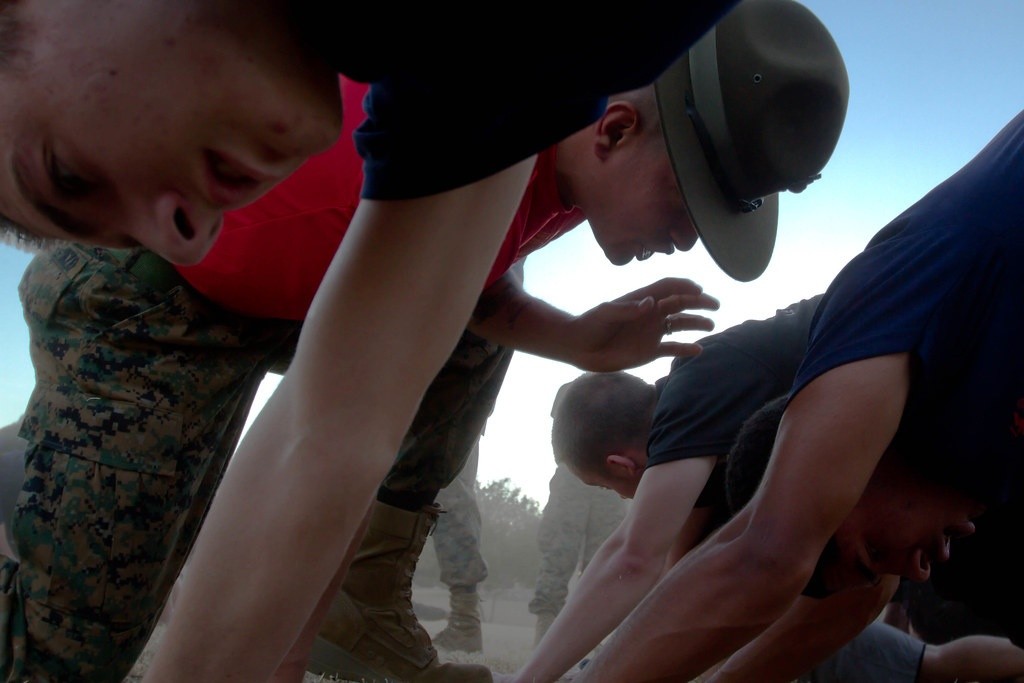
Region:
[0,1,1024,683]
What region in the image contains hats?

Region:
[653,0,850,282]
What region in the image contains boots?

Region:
[534,615,552,645]
[306,498,493,683]
[431,592,481,653]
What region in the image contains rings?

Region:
[666,314,672,334]
